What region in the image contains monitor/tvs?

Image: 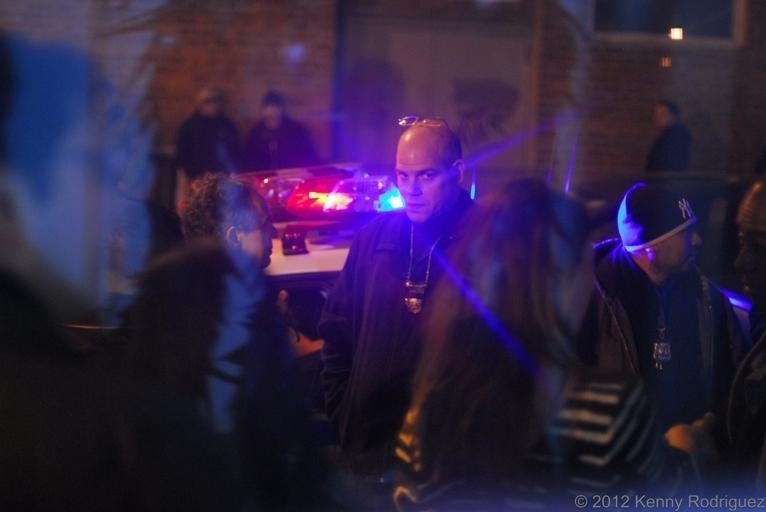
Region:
[579,1,751,49]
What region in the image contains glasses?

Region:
[398,115,451,132]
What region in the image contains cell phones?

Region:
[282,238,308,255]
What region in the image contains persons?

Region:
[0,27,765,511]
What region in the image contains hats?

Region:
[616,183,696,255]
